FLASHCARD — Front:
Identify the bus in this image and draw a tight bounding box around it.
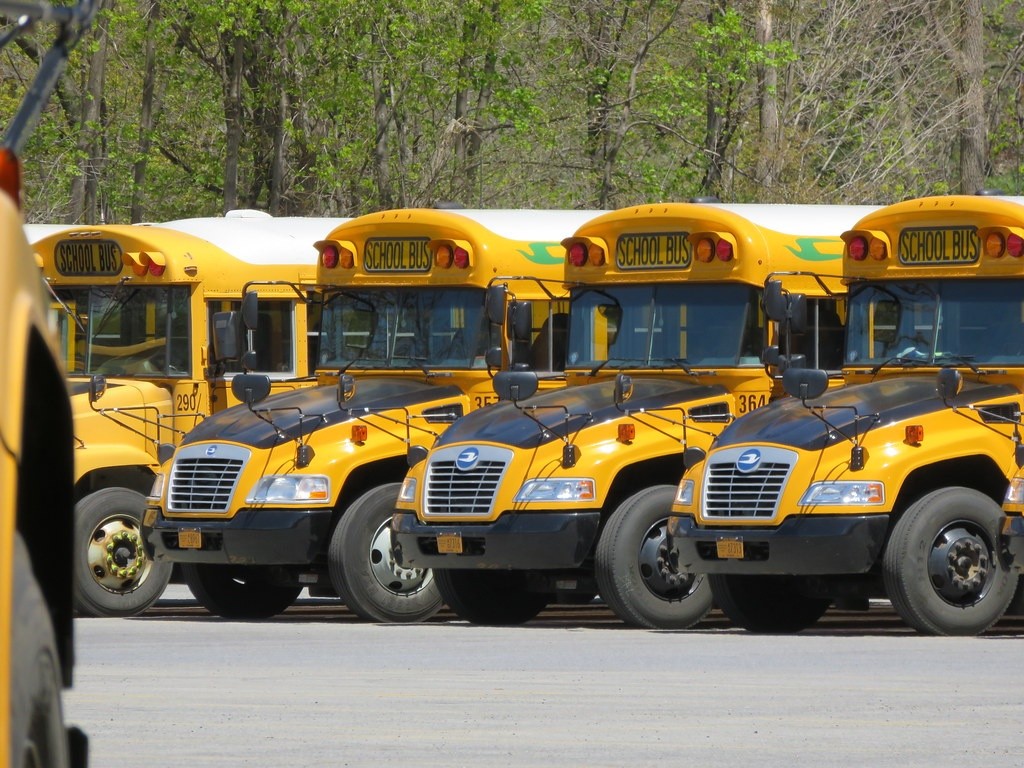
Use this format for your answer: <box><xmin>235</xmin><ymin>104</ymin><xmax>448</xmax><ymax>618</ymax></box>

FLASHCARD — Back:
<box><xmin>0</xmin><ymin>0</ymin><xmax>103</xmax><ymax>768</ymax></box>
<box><xmin>22</xmin><ymin>209</ymin><xmax>355</xmax><ymax>616</ymax></box>
<box><xmin>612</xmin><ymin>188</ymin><xmax>1024</xmax><ymax>637</ymax></box>
<box><xmin>936</xmin><ymin>367</ymin><xmax>1024</xmax><ymax>538</ymax></box>
<box><xmin>336</xmin><ymin>197</ymin><xmax>891</xmax><ymax>632</ymax></box>
<box><xmin>89</xmin><ymin>200</ymin><xmax>614</xmax><ymax>625</ymax></box>
